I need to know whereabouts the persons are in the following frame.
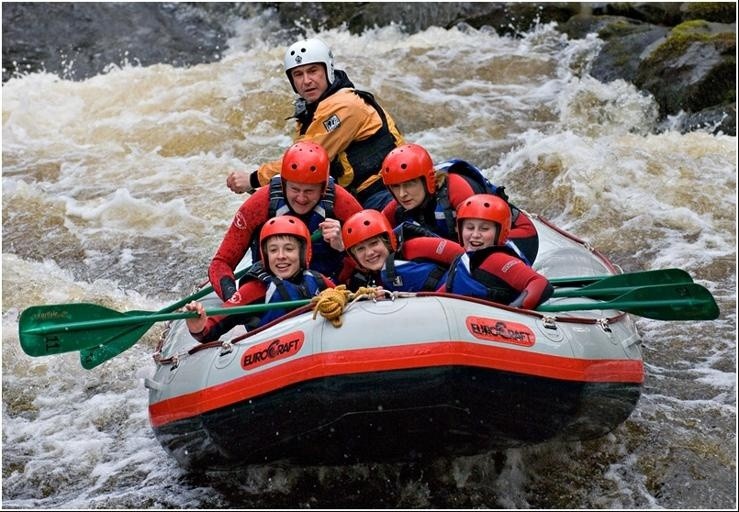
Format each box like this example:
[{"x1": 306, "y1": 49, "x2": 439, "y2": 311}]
[
  {"x1": 434, "y1": 194, "x2": 554, "y2": 311},
  {"x1": 181, "y1": 216, "x2": 338, "y2": 344},
  {"x1": 208, "y1": 142, "x2": 364, "y2": 302},
  {"x1": 341, "y1": 210, "x2": 447, "y2": 292},
  {"x1": 226, "y1": 38, "x2": 402, "y2": 214},
  {"x1": 379, "y1": 144, "x2": 538, "y2": 267}
]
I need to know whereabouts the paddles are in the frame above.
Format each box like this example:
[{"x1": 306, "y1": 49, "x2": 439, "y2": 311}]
[
  {"x1": 80, "y1": 231, "x2": 320, "y2": 370},
  {"x1": 534, "y1": 283, "x2": 721, "y2": 321},
  {"x1": 18, "y1": 299, "x2": 308, "y2": 357},
  {"x1": 551, "y1": 269, "x2": 693, "y2": 301}
]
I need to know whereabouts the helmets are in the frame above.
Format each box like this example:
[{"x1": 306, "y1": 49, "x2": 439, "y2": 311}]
[
  {"x1": 343, "y1": 209, "x2": 398, "y2": 270},
  {"x1": 456, "y1": 194, "x2": 512, "y2": 246},
  {"x1": 382, "y1": 144, "x2": 436, "y2": 201},
  {"x1": 281, "y1": 142, "x2": 330, "y2": 198},
  {"x1": 259, "y1": 216, "x2": 314, "y2": 270},
  {"x1": 285, "y1": 39, "x2": 335, "y2": 94}
]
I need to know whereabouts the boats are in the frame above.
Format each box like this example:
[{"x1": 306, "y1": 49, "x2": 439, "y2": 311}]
[{"x1": 145, "y1": 207, "x2": 644, "y2": 471}]
